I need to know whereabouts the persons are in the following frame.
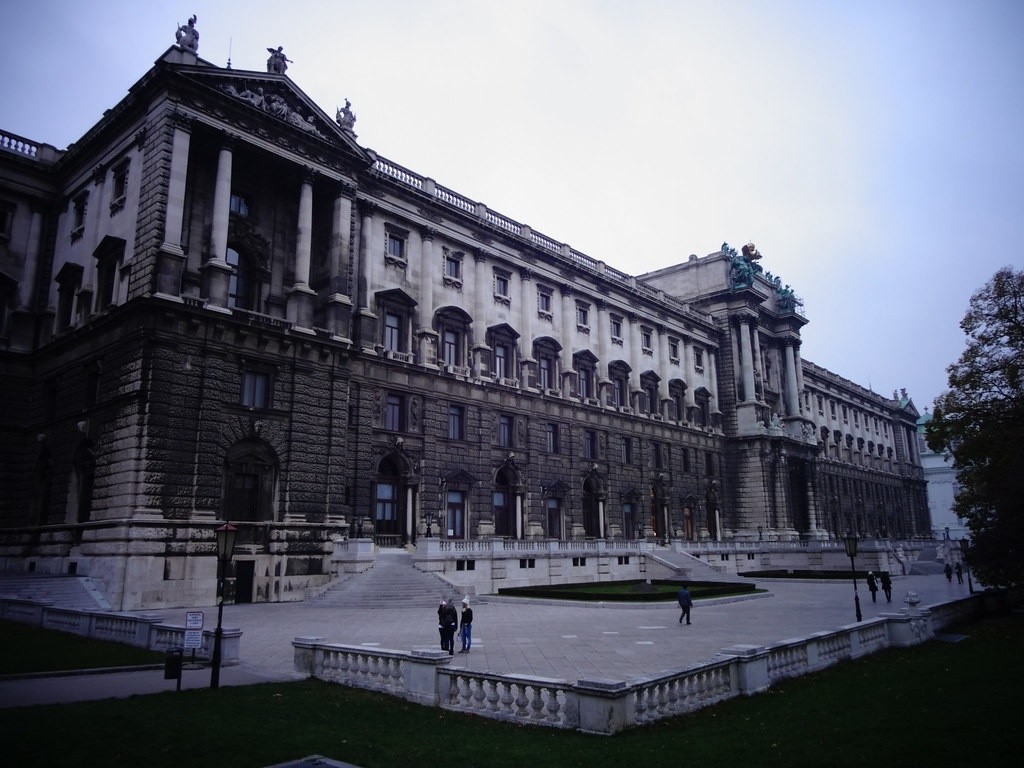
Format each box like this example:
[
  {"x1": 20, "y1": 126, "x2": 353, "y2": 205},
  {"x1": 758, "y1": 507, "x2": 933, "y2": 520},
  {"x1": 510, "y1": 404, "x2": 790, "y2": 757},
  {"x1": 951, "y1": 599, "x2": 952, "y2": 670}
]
[
  {"x1": 881, "y1": 571, "x2": 892, "y2": 603},
  {"x1": 773, "y1": 285, "x2": 802, "y2": 311},
  {"x1": 944, "y1": 564, "x2": 952, "y2": 582},
  {"x1": 337, "y1": 97, "x2": 356, "y2": 128},
  {"x1": 176, "y1": 14, "x2": 199, "y2": 51},
  {"x1": 678, "y1": 584, "x2": 693, "y2": 625},
  {"x1": 867, "y1": 571, "x2": 879, "y2": 603},
  {"x1": 954, "y1": 562, "x2": 963, "y2": 584},
  {"x1": 266, "y1": 46, "x2": 293, "y2": 74},
  {"x1": 729, "y1": 257, "x2": 759, "y2": 285},
  {"x1": 239, "y1": 88, "x2": 320, "y2": 135},
  {"x1": 437, "y1": 598, "x2": 458, "y2": 655},
  {"x1": 458, "y1": 597, "x2": 473, "y2": 653},
  {"x1": 893, "y1": 389, "x2": 899, "y2": 400}
]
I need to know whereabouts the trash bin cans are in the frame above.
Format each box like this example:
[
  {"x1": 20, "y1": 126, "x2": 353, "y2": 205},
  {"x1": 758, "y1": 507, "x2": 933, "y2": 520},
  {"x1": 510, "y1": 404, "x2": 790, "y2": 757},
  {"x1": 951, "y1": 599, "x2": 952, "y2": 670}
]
[{"x1": 163, "y1": 646, "x2": 184, "y2": 680}]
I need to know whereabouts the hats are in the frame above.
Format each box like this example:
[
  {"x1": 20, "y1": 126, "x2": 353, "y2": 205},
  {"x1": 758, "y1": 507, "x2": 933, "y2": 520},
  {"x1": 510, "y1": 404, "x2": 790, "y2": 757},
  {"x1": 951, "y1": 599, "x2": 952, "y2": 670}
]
[
  {"x1": 462, "y1": 596, "x2": 469, "y2": 604},
  {"x1": 440, "y1": 599, "x2": 446, "y2": 605},
  {"x1": 447, "y1": 598, "x2": 452, "y2": 606},
  {"x1": 868, "y1": 570, "x2": 873, "y2": 575}
]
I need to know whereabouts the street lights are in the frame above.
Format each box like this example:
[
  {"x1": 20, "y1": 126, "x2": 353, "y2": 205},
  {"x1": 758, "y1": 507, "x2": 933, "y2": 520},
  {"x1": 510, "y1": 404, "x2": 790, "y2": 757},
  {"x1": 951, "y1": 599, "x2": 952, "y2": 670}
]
[
  {"x1": 840, "y1": 533, "x2": 872, "y2": 622},
  {"x1": 955, "y1": 535, "x2": 980, "y2": 594},
  {"x1": 210, "y1": 518, "x2": 238, "y2": 691}
]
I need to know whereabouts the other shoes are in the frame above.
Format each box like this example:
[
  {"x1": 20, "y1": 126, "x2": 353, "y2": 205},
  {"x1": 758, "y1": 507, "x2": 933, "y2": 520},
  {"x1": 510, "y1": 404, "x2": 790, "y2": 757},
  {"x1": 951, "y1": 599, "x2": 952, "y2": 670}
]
[
  {"x1": 458, "y1": 650, "x2": 470, "y2": 653},
  {"x1": 680, "y1": 621, "x2": 682, "y2": 623},
  {"x1": 887, "y1": 600, "x2": 892, "y2": 603},
  {"x1": 687, "y1": 623, "x2": 691, "y2": 624}
]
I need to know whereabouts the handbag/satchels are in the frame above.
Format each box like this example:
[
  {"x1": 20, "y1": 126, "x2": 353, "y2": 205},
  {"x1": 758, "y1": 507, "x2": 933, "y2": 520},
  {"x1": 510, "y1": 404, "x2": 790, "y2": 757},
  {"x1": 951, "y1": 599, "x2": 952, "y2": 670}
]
[
  {"x1": 457, "y1": 633, "x2": 462, "y2": 642},
  {"x1": 874, "y1": 580, "x2": 880, "y2": 589}
]
